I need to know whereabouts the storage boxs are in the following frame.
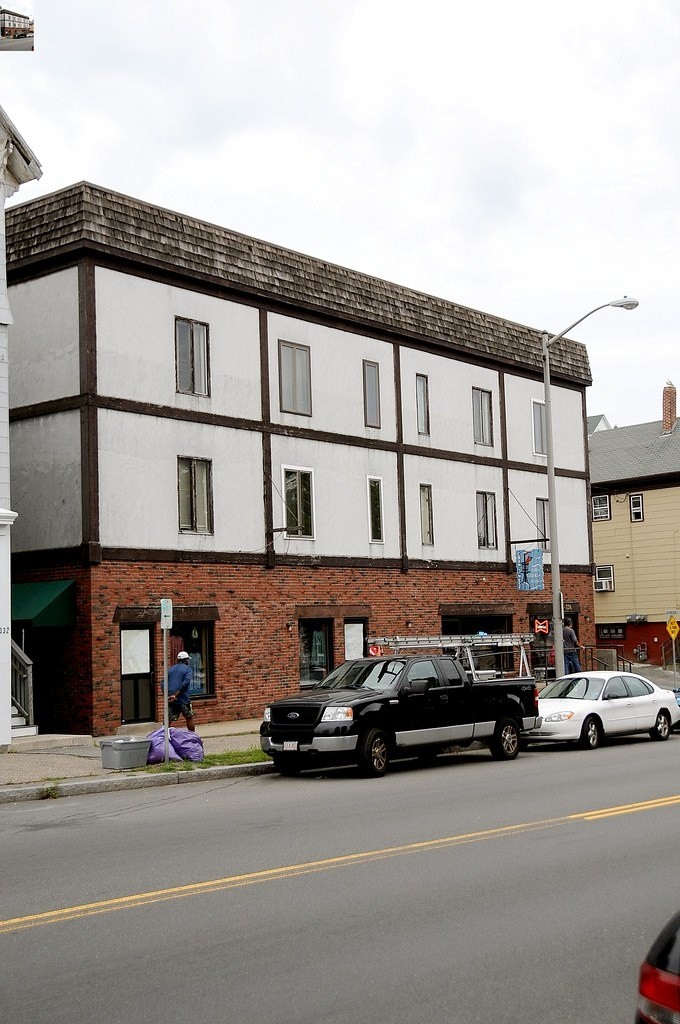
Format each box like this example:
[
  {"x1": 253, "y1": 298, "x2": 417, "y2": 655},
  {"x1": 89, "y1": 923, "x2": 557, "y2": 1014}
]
[{"x1": 99, "y1": 735, "x2": 153, "y2": 769}]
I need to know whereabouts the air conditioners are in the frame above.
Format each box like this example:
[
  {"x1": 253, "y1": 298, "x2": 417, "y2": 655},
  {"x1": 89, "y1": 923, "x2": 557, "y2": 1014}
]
[{"x1": 593, "y1": 580, "x2": 609, "y2": 590}]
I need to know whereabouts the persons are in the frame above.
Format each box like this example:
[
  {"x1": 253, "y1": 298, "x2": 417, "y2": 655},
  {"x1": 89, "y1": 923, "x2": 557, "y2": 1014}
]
[
  {"x1": 161, "y1": 651, "x2": 196, "y2": 734},
  {"x1": 563, "y1": 617, "x2": 585, "y2": 676}
]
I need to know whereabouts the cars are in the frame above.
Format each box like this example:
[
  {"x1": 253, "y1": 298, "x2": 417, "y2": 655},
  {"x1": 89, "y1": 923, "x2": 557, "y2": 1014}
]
[{"x1": 525, "y1": 669, "x2": 680, "y2": 750}]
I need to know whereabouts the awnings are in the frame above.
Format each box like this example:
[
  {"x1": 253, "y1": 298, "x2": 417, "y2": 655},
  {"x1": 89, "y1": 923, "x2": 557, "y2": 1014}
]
[{"x1": 12, "y1": 579, "x2": 78, "y2": 628}]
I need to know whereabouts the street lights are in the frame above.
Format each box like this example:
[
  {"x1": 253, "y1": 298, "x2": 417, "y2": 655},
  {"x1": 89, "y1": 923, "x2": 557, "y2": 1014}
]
[{"x1": 539, "y1": 297, "x2": 639, "y2": 679}]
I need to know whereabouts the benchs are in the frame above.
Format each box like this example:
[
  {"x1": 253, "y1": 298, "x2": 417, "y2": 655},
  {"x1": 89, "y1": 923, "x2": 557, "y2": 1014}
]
[
  {"x1": 534, "y1": 667, "x2": 555, "y2": 681},
  {"x1": 495, "y1": 671, "x2": 507, "y2": 679}
]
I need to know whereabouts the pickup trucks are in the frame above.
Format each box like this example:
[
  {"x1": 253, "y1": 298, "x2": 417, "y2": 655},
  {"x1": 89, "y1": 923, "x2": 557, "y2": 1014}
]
[{"x1": 259, "y1": 632, "x2": 544, "y2": 780}]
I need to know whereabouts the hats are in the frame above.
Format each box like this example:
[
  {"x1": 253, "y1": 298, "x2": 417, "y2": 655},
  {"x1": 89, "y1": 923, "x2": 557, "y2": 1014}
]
[{"x1": 177, "y1": 651, "x2": 192, "y2": 659}]
